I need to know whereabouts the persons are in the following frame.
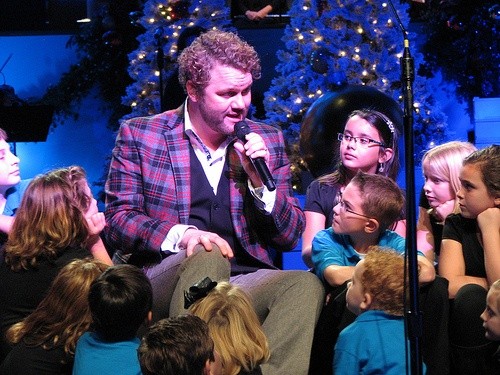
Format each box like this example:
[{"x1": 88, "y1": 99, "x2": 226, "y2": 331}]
[
  {"x1": 245, "y1": 0, "x2": 282, "y2": 20},
  {"x1": 0, "y1": 28, "x2": 500, "y2": 375}
]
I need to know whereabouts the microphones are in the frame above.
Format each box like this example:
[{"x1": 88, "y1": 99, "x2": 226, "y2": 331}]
[{"x1": 234, "y1": 120, "x2": 276, "y2": 192}]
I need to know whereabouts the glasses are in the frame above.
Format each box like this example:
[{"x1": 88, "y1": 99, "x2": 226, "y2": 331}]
[
  {"x1": 337, "y1": 132, "x2": 384, "y2": 147},
  {"x1": 336, "y1": 191, "x2": 371, "y2": 220}
]
